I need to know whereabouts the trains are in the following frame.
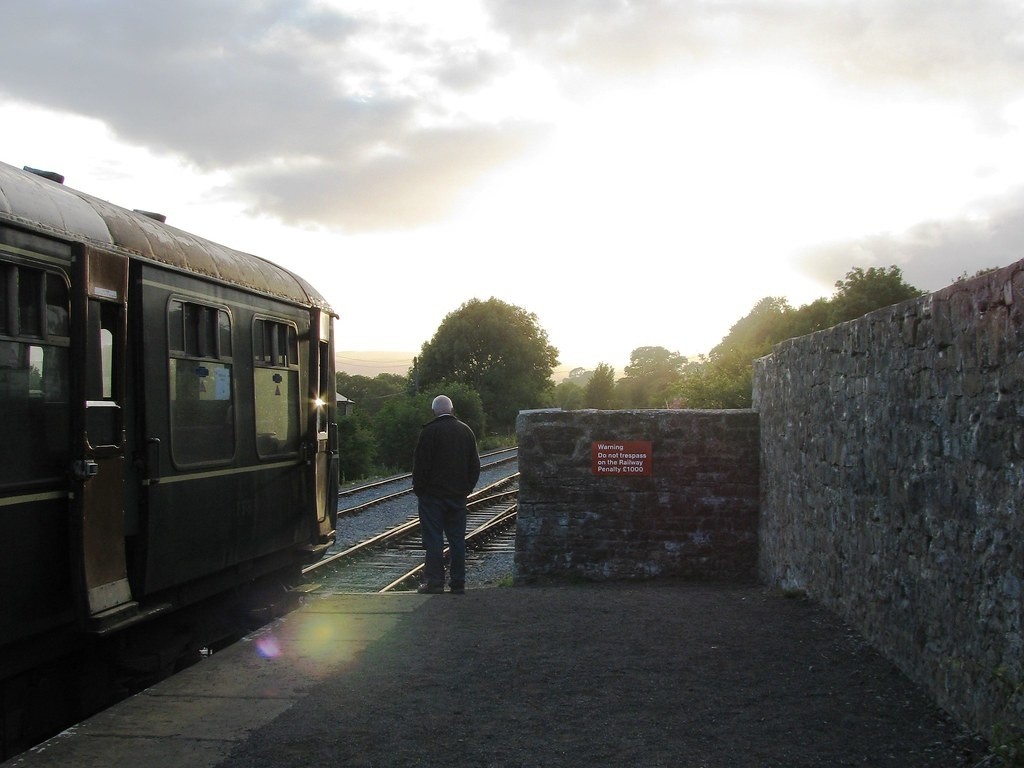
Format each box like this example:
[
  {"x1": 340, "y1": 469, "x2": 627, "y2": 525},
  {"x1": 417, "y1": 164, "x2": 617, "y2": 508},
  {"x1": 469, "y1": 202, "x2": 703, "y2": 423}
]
[{"x1": 1, "y1": 159, "x2": 357, "y2": 681}]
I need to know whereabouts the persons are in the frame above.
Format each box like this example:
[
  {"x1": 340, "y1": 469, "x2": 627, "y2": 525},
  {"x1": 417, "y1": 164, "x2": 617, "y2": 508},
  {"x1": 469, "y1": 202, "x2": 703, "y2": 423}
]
[{"x1": 412, "y1": 395, "x2": 480, "y2": 594}]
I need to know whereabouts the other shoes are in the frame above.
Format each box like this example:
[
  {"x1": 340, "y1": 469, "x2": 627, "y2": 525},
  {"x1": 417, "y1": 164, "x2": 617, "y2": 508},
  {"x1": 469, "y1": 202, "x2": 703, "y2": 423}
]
[
  {"x1": 449, "y1": 581, "x2": 465, "y2": 594},
  {"x1": 418, "y1": 584, "x2": 444, "y2": 594}
]
